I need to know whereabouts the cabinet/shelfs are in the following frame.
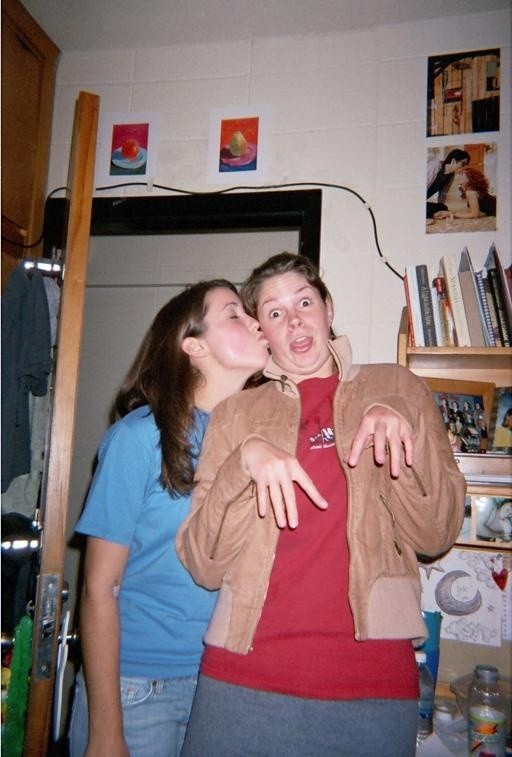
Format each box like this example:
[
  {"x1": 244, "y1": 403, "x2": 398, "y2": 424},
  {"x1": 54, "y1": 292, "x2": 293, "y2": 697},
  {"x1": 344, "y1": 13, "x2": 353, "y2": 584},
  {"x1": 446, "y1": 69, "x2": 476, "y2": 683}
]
[
  {"x1": 1, "y1": 1, "x2": 65, "y2": 295},
  {"x1": 397, "y1": 307, "x2": 512, "y2": 702}
]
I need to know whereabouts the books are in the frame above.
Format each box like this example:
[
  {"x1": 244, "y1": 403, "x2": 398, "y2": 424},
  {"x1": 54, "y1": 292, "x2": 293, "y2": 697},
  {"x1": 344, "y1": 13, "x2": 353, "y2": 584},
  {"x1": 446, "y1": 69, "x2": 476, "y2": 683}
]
[{"x1": 403, "y1": 241, "x2": 512, "y2": 347}]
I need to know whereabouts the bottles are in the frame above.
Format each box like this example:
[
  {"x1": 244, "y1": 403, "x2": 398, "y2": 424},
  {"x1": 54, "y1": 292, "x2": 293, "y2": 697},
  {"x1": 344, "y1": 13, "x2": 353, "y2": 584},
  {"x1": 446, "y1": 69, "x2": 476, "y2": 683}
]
[
  {"x1": 416, "y1": 651, "x2": 435, "y2": 747},
  {"x1": 468, "y1": 664, "x2": 506, "y2": 757}
]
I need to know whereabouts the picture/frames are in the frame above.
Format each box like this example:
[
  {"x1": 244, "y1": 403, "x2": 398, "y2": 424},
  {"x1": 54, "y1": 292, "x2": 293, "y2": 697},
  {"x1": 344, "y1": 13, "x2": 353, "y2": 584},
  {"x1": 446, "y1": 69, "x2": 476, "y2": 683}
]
[
  {"x1": 486, "y1": 387, "x2": 512, "y2": 451},
  {"x1": 419, "y1": 377, "x2": 495, "y2": 453}
]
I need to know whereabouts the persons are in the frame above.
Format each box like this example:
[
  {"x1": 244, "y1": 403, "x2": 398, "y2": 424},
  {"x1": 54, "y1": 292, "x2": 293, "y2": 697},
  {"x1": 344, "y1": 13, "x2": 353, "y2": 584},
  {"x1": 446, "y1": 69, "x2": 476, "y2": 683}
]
[
  {"x1": 439, "y1": 398, "x2": 487, "y2": 453},
  {"x1": 69, "y1": 279, "x2": 270, "y2": 757},
  {"x1": 435, "y1": 167, "x2": 496, "y2": 218},
  {"x1": 426, "y1": 149, "x2": 471, "y2": 219},
  {"x1": 175, "y1": 250, "x2": 466, "y2": 757},
  {"x1": 475, "y1": 497, "x2": 512, "y2": 542}
]
